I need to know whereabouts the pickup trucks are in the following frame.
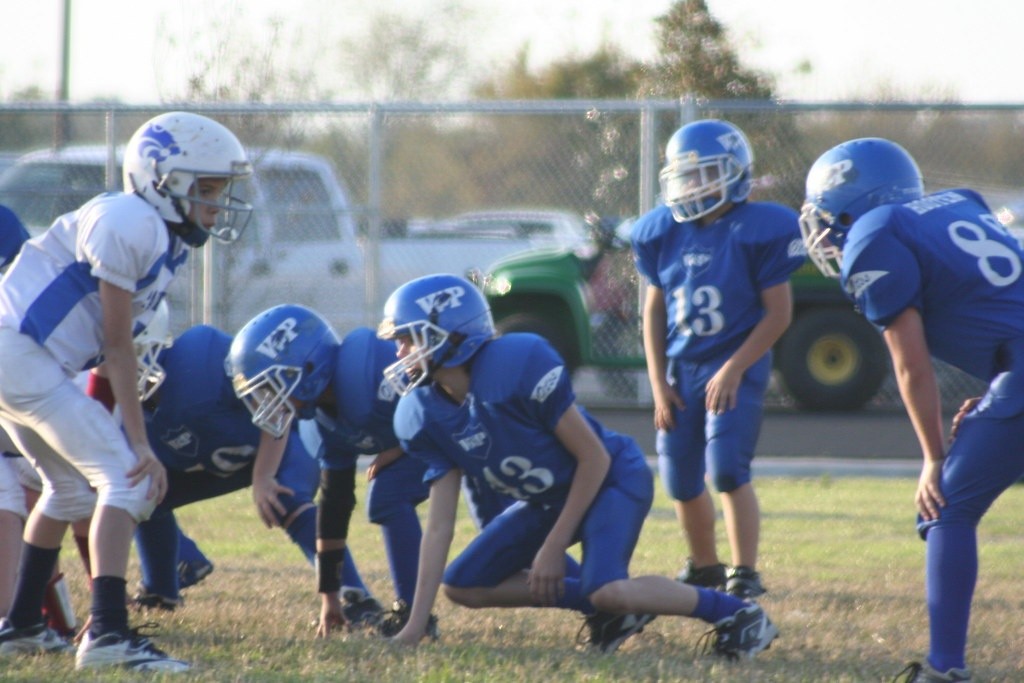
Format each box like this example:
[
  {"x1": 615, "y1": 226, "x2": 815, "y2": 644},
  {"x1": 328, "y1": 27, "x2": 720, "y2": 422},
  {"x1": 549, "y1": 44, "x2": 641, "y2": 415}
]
[{"x1": 0, "y1": 146, "x2": 589, "y2": 353}]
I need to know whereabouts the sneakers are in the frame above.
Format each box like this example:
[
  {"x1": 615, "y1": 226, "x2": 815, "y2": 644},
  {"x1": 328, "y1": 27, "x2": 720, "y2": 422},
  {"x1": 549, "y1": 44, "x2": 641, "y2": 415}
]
[
  {"x1": 73, "y1": 626, "x2": 192, "y2": 675},
  {"x1": 575, "y1": 609, "x2": 656, "y2": 659},
  {"x1": 335, "y1": 595, "x2": 382, "y2": 631},
  {"x1": 724, "y1": 564, "x2": 763, "y2": 598},
  {"x1": 366, "y1": 603, "x2": 437, "y2": 642},
  {"x1": 178, "y1": 556, "x2": 213, "y2": 588},
  {"x1": 128, "y1": 580, "x2": 182, "y2": 615},
  {"x1": 693, "y1": 598, "x2": 779, "y2": 668},
  {"x1": 674, "y1": 558, "x2": 728, "y2": 587},
  {"x1": 0, "y1": 613, "x2": 69, "y2": 656}
]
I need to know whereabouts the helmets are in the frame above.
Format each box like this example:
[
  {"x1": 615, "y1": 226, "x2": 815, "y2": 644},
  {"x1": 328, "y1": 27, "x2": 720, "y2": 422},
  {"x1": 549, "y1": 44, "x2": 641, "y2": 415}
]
[
  {"x1": 93, "y1": 296, "x2": 171, "y2": 402},
  {"x1": 657, "y1": 118, "x2": 753, "y2": 222},
  {"x1": 798, "y1": 137, "x2": 924, "y2": 276},
  {"x1": 223, "y1": 303, "x2": 341, "y2": 440},
  {"x1": 377, "y1": 273, "x2": 497, "y2": 398},
  {"x1": 123, "y1": 113, "x2": 255, "y2": 247}
]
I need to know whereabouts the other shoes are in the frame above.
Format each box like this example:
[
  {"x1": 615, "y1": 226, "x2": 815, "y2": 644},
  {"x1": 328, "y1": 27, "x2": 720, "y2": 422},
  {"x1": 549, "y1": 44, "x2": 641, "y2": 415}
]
[{"x1": 889, "y1": 661, "x2": 969, "y2": 683}]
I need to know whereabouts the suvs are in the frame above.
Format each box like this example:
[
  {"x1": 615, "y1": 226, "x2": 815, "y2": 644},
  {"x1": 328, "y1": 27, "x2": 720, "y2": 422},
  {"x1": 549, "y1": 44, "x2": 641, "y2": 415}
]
[{"x1": 465, "y1": 207, "x2": 895, "y2": 416}]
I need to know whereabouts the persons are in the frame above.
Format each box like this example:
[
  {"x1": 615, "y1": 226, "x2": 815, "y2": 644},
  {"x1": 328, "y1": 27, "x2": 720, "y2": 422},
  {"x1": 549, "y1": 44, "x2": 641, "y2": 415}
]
[
  {"x1": 0, "y1": 113, "x2": 255, "y2": 672},
  {"x1": 630, "y1": 120, "x2": 806, "y2": 599},
  {"x1": 377, "y1": 273, "x2": 780, "y2": 672},
  {"x1": 797, "y1": 137, "x2": 1024, "y2": 683},
  {"x1": 224, "y1": 304, "x2": 583, "y2": 643},
  {"x1": 128, "y1": 295, "x2": 383, "y2": 633}
]
